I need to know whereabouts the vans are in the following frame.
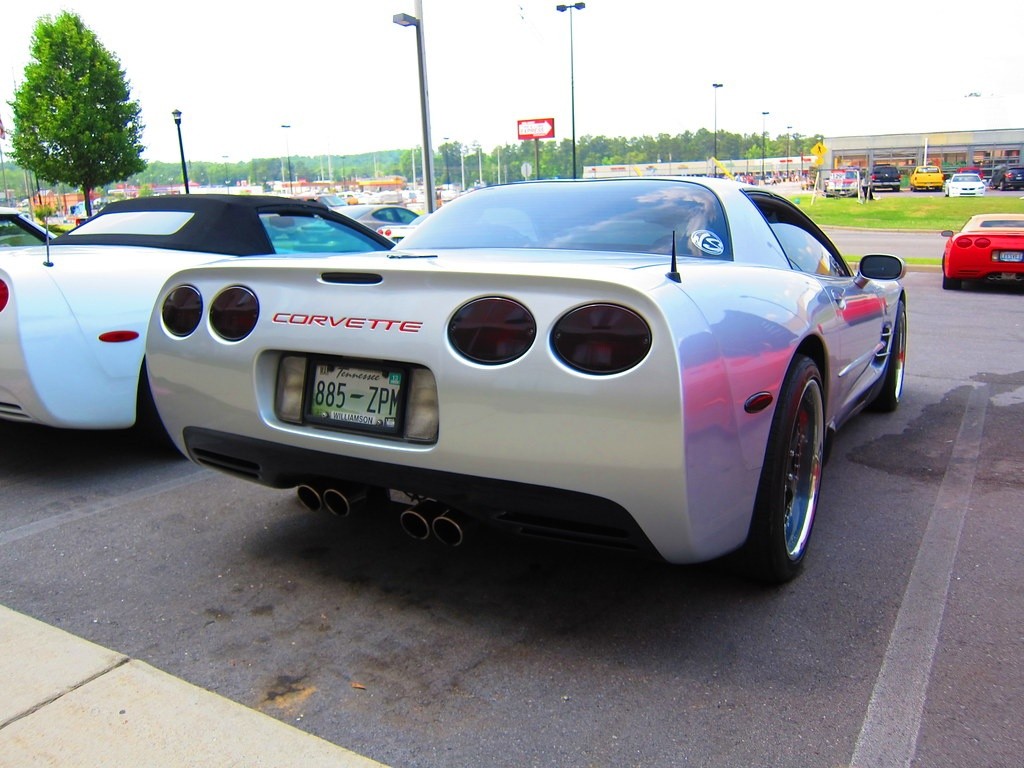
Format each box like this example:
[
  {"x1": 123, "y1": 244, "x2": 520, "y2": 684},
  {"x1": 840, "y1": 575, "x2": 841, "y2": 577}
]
[{"x1": 957, "y1": 164, "x2": 984, "y2": 182}]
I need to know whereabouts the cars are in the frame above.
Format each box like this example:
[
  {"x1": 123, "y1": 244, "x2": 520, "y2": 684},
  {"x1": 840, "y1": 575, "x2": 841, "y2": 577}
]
[
  {"x1": 0, "y1": 167, "x2": 472, "y2": 279},
  {"x1": 824, "y1": 167, "x2": 863, "y2": 197},
  {"x1": 910, "y1": 165, "x2": 945, "y2": 192},
  {"x1": 989, "y1": 163, "x2": 1024, "y2": 191},
  {"x1": 944, "y1": 173, "x2": 986, "y2": 199}
]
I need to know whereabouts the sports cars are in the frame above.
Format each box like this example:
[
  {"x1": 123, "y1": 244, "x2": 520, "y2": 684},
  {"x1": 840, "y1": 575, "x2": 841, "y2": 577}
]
[
  {"x1": 0, "y1": 191, "x2": 396, "y2": 435},
  {"x1": 941, "y1": 214, "x2": 1024, "y2": 290},
  {"x1": 147, "y1": 172, "x2": 908, "y2": 589}
]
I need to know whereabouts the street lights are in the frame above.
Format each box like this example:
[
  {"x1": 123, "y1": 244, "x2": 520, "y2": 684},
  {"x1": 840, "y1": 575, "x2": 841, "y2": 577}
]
[
  {"x1": 171, "y1": 108, "x2": 190, "y2": 194},
  {"x1": 392, "y1": 0, "x2": 437, "y2": 215},
  {"x1": 761, "y1": 112, "x2": 770, "y2": 180},
  {"x1": 712, "y1": 83, "x2": 724, "y2": 178},
  {"x1": 557, "y1": 2, "x2": 587, "y2": 179}
]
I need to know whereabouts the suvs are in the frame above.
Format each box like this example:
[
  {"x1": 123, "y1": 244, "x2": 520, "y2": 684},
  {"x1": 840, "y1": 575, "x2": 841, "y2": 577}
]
[{"x1": 870, "y1": 166, "x2": 901, "y2": 192}]
